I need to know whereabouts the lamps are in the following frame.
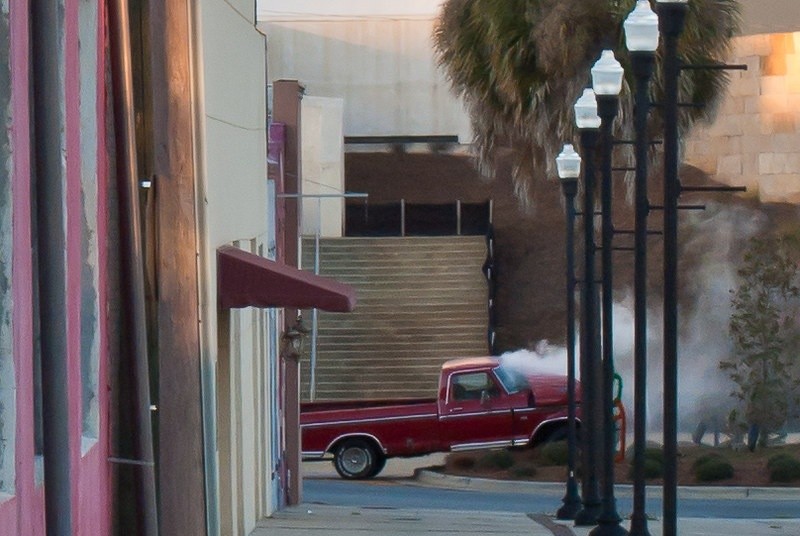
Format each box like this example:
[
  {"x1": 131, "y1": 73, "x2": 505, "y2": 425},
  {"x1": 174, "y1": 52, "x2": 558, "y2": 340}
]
[{"x1": 281, "y1": 316, "x2": 312, "y2": 367}]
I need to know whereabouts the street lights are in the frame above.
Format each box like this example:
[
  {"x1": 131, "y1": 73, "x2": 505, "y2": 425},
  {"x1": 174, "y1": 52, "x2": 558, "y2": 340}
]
[
  {"x1": 589, "y1": 48, "x2": 630, "y2": 536},
  {"x1": 623, "y1": 0, "x2": 660, "y2": 536},
  {"x1": 555, "y1": 142, "x2": 584, "y2": 522},
  {"x1": 574, "y1": 86, "x2": 602, "y2": 527}
]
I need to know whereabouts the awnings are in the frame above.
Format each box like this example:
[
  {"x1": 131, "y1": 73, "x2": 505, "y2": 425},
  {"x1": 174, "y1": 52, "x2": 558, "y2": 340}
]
[{"x1": 216, "y1": 244, "x2": 357, "y2": 313}]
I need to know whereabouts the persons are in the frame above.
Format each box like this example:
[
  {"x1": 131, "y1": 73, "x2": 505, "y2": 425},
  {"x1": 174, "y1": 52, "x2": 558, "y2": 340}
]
[{"x1": 748, "y1": 417, "x2": 760, "y2": 451}]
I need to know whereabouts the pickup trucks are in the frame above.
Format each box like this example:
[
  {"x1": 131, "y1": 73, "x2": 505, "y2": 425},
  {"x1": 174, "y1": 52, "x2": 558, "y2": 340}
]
[{"x1": 299, "y1": 356, "x2": 581, "y2": 482}]
